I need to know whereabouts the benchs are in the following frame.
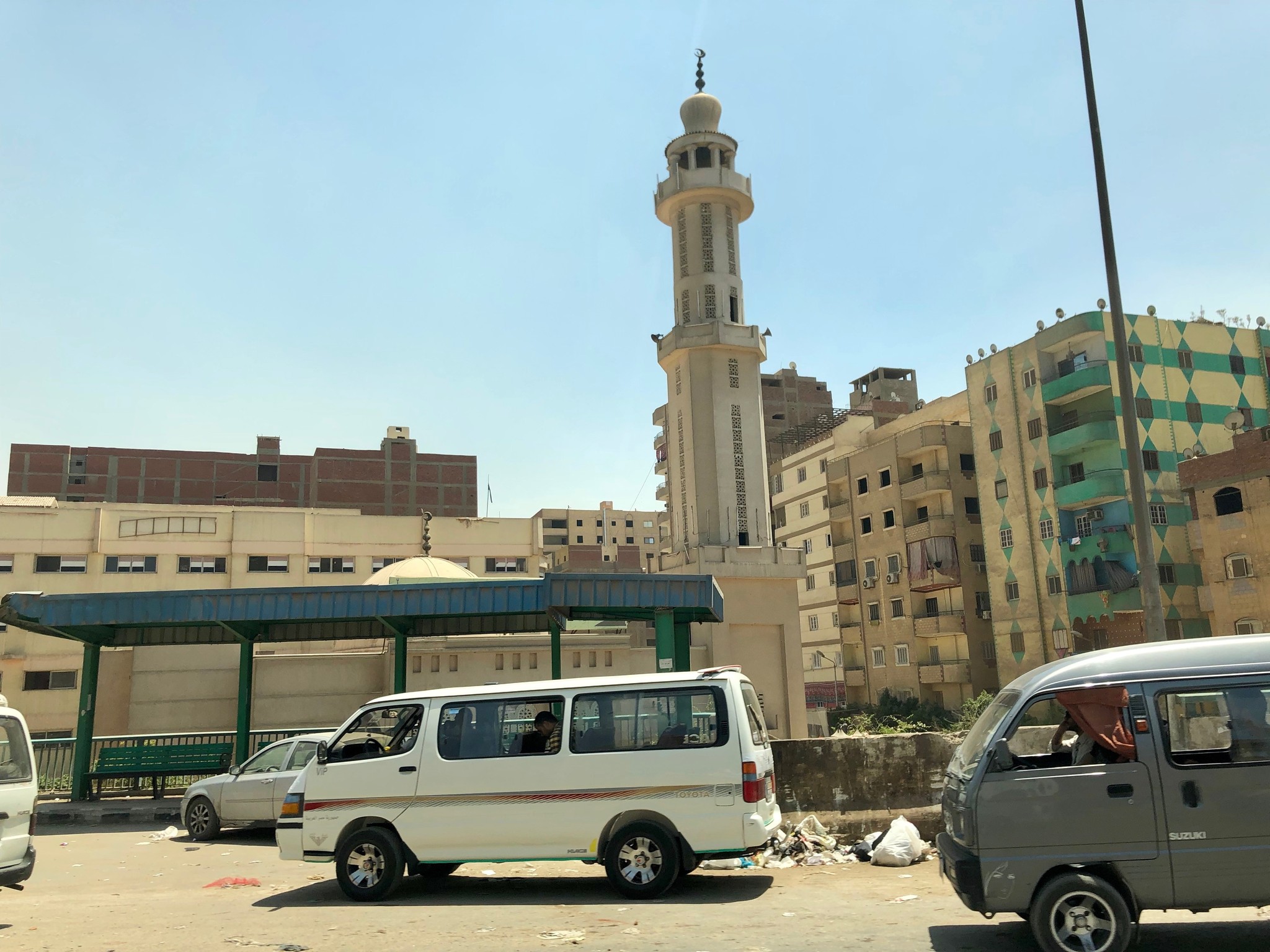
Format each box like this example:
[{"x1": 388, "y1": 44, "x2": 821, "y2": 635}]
[
  {"x1": 254, "y1": 741, "x2": 317, "y2": 768},
  {"x1": 83, "y1": 742, "x2": 235, "y2": 803}
]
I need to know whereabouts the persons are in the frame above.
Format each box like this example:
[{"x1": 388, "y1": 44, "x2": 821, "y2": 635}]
[
  {"x1": 1052, "y1": 710, "x2": 1096, "y2": 766},
  {"x1": 534, "y1": 711, "x2": 562, "y2": 752},
  {"x1": 444, "y1": 707, "x2": 480, "y2": 746}
]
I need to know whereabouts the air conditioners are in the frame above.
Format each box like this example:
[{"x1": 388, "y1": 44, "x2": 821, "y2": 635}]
[
  {"x1": 885, "y1": 573, "x2": 898, "y2": 584},
  {"x1": 862, "y1": 578, "x2": 875, "y2": 590},
  {"x1": 975, "y1": 564, "x2": 986, "y2": 573},
  {"x1": 1086, "y1": 510, "x2": 1104, "y2": 521},
  {"x1": 983, "y1": 611, "x2": 992, "y2": 620}
]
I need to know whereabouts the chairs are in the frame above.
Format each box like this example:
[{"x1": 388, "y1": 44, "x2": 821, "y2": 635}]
[
  {"x1": 600, "y1": 726, "x2": 617, "y2": 751},
  {"x1": 506, "y1": 729, "x2": 541, "y2": 755},
  {"x1": 655, "y1": 723, "x2": 688, "y2": 748},
  {"x1": 687, "y1": 725, "x2": 703, "y2": 744},
  {"x1": 441, "y1": 721, "x2": 480, "y2": 758},
  {"x1": 575, "y1": 726, "x2": 603, "y2": 751}
]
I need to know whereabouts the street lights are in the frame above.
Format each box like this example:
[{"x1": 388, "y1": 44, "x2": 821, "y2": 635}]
[{"x1": 816, "y1": 649, "x2": 838, "y2": 708}]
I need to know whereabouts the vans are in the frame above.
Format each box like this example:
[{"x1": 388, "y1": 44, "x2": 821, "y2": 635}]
[
  {"x1": 0, "y1": 694, "x2": 40, "y2": 892},
  {"x1": 934, "y1": 631, "x2": 1270, "y2": 952},
  {"x1": 275, "y1": 663, "x2": 785, "y2": 903}
]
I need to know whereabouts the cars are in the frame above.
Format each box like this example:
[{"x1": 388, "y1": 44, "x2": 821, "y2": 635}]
[{"x1": 179, "y1": 730, "x2": 395, "y2": 841}]
